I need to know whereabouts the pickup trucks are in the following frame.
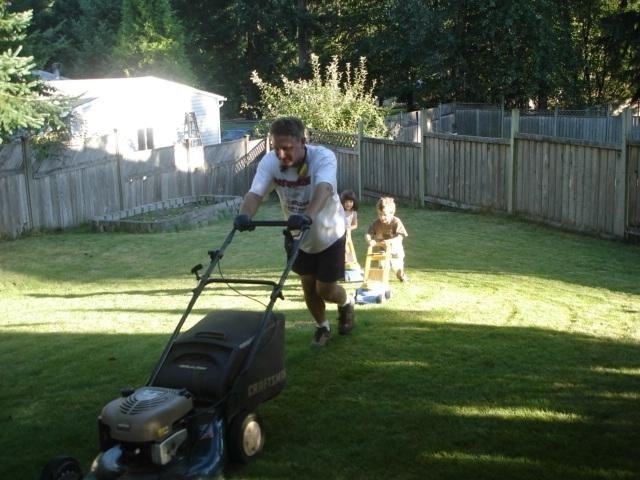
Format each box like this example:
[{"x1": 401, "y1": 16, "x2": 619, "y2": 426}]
[{"x1": 221, "y1": 128, "x2": 263, "y2": 142}]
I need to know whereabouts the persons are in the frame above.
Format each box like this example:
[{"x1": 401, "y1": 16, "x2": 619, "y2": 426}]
[
  {"x1": 339, "y1": 188, "x2": 358, "y2": 260},
  {"x1": 364, "y1": 197, "x2": 408, "y2": 281},
  {"x1": 234, "y1": 116, "x2": 355, "y2": 349}
]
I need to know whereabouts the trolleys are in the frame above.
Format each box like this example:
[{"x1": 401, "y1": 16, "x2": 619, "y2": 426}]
[{"x1": 40, "y1": 216, "x2": 314, "y2": 479}]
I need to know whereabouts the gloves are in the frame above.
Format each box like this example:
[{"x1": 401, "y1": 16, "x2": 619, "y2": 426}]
[
  {"x1": 287, "y1": 213, "x2": 313, "y2": 231},
  {"x1": 233, "y1": 214, "x2": 256, "y2": 232}
]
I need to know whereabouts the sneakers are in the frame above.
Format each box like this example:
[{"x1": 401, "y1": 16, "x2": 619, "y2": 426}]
[
  {"x1": 396, "y1": 269, "x2": 406, "y2": 281},
  {"x1": 309, "y1": 325, "x2": 332, "y2": 349},
  {"x1": 337, "y1": 293, "x2": 356, "y2": 336}
]
